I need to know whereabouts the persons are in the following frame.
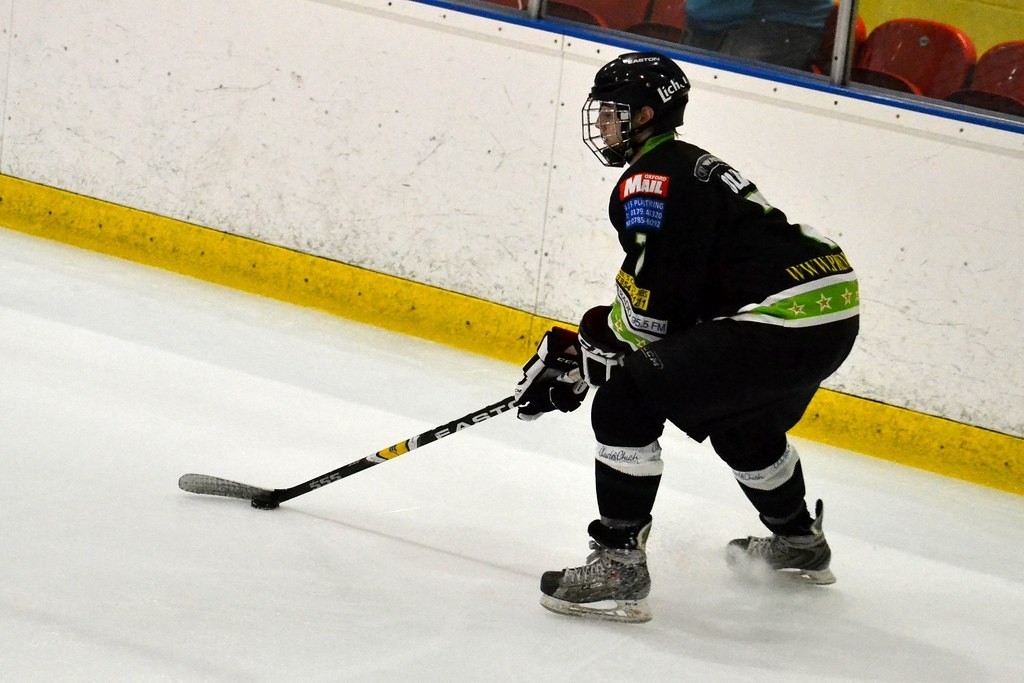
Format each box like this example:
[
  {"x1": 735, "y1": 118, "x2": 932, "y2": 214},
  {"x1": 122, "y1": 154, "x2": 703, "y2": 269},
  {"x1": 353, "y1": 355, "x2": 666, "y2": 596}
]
[
  {"x1": 683, "y1": 0, "x2": 834, "y2": 71},
  {"x1": 514, "y1": 50, "x2": 862, "y2": 623}
]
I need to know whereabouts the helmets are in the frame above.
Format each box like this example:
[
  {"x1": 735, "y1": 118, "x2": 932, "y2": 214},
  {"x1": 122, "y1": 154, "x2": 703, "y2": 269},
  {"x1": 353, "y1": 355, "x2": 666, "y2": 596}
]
[{"x1": 582, "y1": 52, "x2": 691, "y2": 167}]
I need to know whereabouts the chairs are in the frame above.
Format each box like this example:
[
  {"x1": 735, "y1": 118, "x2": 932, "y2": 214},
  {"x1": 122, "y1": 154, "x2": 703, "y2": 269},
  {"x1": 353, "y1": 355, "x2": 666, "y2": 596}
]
[
  {"x1": 971, "y1": 40, "x2": 1024, "y2": 103},
  {"x1": 563, "y1": 0, "x2": 649, "y2": 32},
  {"x1": 850, "y1": 66, "x2": 922, "y2": 96},
  {"x1": 858, "y1": 16, "x2": 976, "y2": 99},
  {"x1": 944, "y1": 90, "x2": 1024, "y2": 117}
]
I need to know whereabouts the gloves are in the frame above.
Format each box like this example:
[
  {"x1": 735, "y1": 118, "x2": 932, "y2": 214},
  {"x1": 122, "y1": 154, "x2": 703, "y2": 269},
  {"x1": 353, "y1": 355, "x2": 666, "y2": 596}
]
[
  {"x1": 579, "y1": 305, "x2": 628, "y2": 386},
  {"x1": 515, "y1": 327, "x2": 590, "y2": 420}
]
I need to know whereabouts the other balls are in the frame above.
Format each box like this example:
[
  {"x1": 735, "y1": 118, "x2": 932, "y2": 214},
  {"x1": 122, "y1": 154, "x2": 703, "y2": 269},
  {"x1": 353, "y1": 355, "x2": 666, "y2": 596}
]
[{"x1": 251, "y1": 496, "x2": 280, "y2": 510}]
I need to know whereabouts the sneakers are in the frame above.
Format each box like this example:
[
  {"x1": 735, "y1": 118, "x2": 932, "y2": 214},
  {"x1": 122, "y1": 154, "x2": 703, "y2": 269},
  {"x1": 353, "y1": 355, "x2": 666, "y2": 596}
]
[
  {"x1": 539, "y1": 514, "x2": 654, "y2": 624},
  {"x1": 723, "y1": 498, "x2": 837, "y2": 586}
]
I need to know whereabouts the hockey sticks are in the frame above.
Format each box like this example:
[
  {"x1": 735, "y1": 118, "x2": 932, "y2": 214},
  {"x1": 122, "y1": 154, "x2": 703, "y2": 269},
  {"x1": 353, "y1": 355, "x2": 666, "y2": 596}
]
[{"x1": 178, "y1": 358, "x2": 585, "y2": 505}]
